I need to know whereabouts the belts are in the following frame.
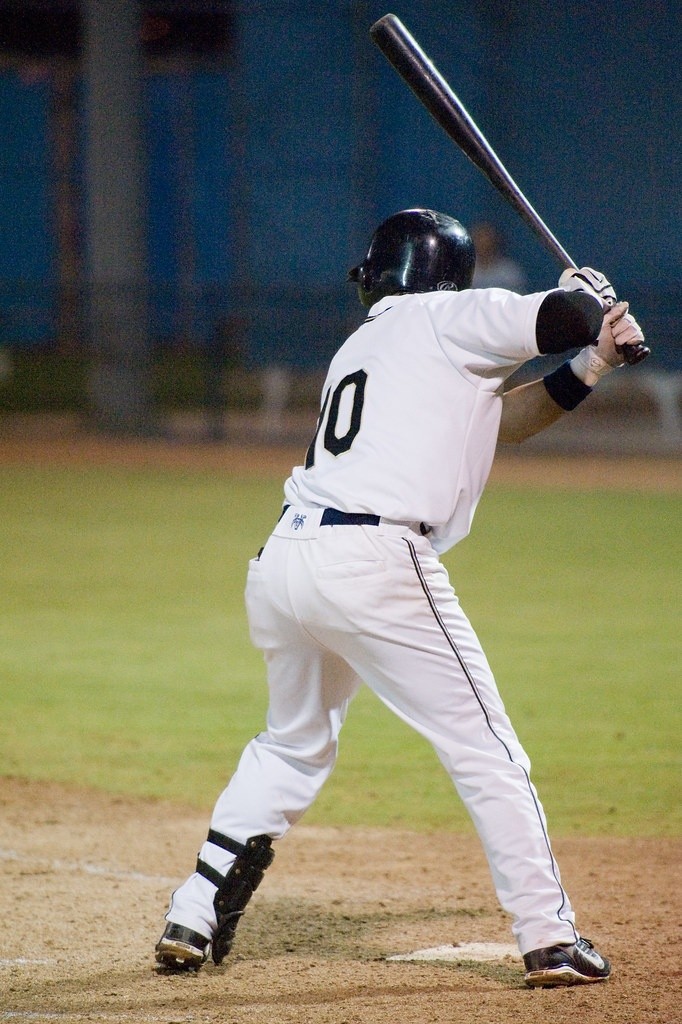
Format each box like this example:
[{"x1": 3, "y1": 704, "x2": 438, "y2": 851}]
[{"x1": 277, "y1": 505, "x2": 428, "y2": 536}]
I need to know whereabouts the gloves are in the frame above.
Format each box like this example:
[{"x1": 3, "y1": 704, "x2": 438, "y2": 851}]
[
  {"x1": 558, "y1": 265, "x2": 617, "y2": 313},
  {"x1": 594, "y1": 301, "x2": 645, "y2": 368}
]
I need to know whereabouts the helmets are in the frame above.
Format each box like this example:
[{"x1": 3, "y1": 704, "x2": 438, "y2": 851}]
[{"x1": 349, "y1": 207, "x2": 476, "y2": 298}]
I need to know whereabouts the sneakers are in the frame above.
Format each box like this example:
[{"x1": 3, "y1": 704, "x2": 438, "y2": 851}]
[
  {"x1": 523, "y1": 937, "x2": 611, "y2": 987},
  {"x1": 155, "y1": 921, "x2": 211, "y2": 972}
]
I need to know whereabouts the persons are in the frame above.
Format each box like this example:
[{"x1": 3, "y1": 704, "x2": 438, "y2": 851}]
[{"x1": 154, "y1": 208, "x2": 646, "y2": 986}]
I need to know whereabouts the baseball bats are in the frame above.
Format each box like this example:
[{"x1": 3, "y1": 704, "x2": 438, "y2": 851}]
[{"x1": 370, "y1": 9, "x2": 656, "y2": 365}]
